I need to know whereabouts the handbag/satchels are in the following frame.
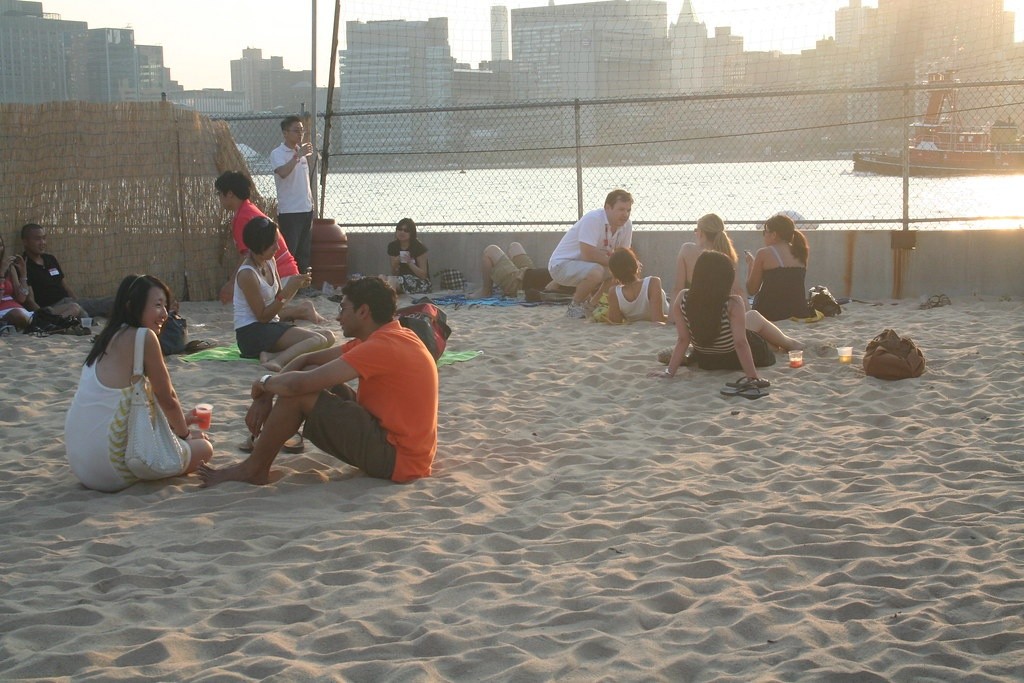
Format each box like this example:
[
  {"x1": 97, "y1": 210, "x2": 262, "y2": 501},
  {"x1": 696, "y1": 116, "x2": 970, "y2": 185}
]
[
  {"x1": 807, "y1": 286, "x2": 841, "y2": 316},
  {"x1": 159, "y1": 301, "x2": 188, "y2": 355},
  {"x1": 863, "y1": 328, "x2": 926, "y2": 380},
  {"x1": 125, "y1": 327, "x2": 186, "y2": 479},
  {"x1": 433, "y1": 269, "x2": 466, "y2": 290}
]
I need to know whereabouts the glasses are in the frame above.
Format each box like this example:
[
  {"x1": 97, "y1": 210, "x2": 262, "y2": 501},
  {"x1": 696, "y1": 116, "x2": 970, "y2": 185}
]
[
  {"x1": 254, "y1": 217, "x2": 273, "y2": 234},
  {"x1": 694, "y1": 228, "x2": 702, "y2": 233},
  {"x1": 396, "y1": 227, "x2": 410, "y2": 233},
  {"x1": 762, "y1": 229, "x2": 766, "y2": 236},
  {"x1": 286, "y1": 127, "x2": 305, "y2": 133}
]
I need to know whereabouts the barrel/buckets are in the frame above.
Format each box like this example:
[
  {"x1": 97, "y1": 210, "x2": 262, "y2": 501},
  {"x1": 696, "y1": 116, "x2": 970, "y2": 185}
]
[{"x1": 311, "y1": 218, "x2": 348, "y2": 289}]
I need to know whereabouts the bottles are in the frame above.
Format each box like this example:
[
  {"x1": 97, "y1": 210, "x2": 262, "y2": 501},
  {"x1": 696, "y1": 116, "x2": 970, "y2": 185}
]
[{"x1": 602, "y1": 245, "x2": 611, "y2": 258}]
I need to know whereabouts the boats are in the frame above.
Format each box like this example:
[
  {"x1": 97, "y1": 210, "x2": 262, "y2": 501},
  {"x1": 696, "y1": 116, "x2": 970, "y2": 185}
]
[{"x1": 848, "y1": 56, "x2": 1024, "y2": 177}]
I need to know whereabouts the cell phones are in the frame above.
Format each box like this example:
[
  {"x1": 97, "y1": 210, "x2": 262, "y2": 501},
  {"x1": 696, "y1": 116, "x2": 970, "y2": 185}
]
[
  {"x1": 744, "y1": 250, "x2": 755, "y2": 261},
  {"x1": 400, "y1": 251, "x2": 409, "y2": 264}
]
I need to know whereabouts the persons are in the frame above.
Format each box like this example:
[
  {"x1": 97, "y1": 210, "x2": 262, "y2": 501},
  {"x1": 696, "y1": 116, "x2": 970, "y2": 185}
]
[
  {"x1": 647, "y1": 249, "x2": 806, "y2": 386},
  {"x1": 466, "y1": 242, "x2": 577, "y2": 303},
  {"x1": 0, "y1": 223, "x2": 98, "y2": 335},
  {"x1": 271, "y1": 116, "x2": 315, "y2": 274},
  {"x1": 379, "y1": 218, "x2": 434, "y2": 296},
  {"x1": 64, "y1": 274, "x2": 214, "y2": 491},
  {"x1": 234, "y1": 217, "x2": 336, "y2": 372},
  {"x1": 608, "y1": 246, "x2": 669, "y2": 323},
  {"x1": 745, "y1": 215, "x2": 808, "y2": 321},
  {"x1": 548, "y1": 189, "x2": 633, "y2": 321},
  {"x1": 216, "y1": 170, "x2": 330, "y2": 325},
  {"x1": 198, "y1": 275, "x2": 439, "y2": 489},
  {"x1": 667, "y1": 213, "x2": 751, "y2": 325}
]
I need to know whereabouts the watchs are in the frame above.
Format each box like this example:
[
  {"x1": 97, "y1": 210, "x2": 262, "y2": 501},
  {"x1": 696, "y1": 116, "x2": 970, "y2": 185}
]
[{"x1": 259, "y1": 374, "x2": 271, "y2": 393}]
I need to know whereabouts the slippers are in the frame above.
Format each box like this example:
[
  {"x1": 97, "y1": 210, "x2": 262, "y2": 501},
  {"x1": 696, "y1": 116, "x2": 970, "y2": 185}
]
[
  {"x1": 238, "y1": 431, "x2": 305, "y2": 454},
  {"x1": 720, "y1": 376, "x2": 770, "y2": 400}
]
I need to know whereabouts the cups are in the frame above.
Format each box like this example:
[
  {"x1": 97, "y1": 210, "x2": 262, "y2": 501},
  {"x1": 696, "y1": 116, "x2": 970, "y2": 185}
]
[
  {"x1": 81, "y1": 318, "x2": 93, "y2": 328},
  {"x1": 517, "y1": 290, "x2": 526, "y2": 301},
  {"x1": 302, "y1": 143, "x2": 312, "y2": 155},
  {"x1": 837, "y1": 347, "x2": 854, "y2": 362},
  {"x1": 788, "y1": 349, "x2": 805, "y2": 368},
  {"x1": 400, "y1": 251, "x2": 409, "y2": 264},
  {"x1": 195, "y1": 403, "x2": 214, "y2": 430}
]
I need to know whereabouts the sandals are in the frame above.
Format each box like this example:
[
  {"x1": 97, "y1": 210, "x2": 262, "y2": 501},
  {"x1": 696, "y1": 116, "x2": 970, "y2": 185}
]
[{"x1": 918, "y1": 294, "x2": 950, "y2": 310}]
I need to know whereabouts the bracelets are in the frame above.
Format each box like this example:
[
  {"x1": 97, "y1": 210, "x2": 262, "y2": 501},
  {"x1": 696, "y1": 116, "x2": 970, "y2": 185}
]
[
  {"x1": 20, "y1": 277, "x2": 28, "y2": 281},
  {"x1": 179, "y1": 429, "x2": 191, "y2": 440},
  {"x1": 18, "y1": 286, "x2": 29, "y2": 296},
  {"x1": 294, "y1": 153, "x2": 301, "y2": 164},
  {"x1": 665, "y1": 368, "x2": 673, "y2": 376},
  {"x1": 275, "y1": 295, "x2": 287, "y2": 304}
]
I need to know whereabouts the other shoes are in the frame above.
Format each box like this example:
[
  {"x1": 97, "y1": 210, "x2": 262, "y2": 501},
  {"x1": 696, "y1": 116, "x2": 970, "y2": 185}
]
[{"x1": 567, "y1": 303, "x2": 586, "y2": 319}]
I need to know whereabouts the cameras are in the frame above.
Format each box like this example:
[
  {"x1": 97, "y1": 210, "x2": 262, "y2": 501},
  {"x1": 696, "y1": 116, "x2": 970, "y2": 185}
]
[{"x1": 8, "y1": 256, "x2": 21, "y2": 264}]
